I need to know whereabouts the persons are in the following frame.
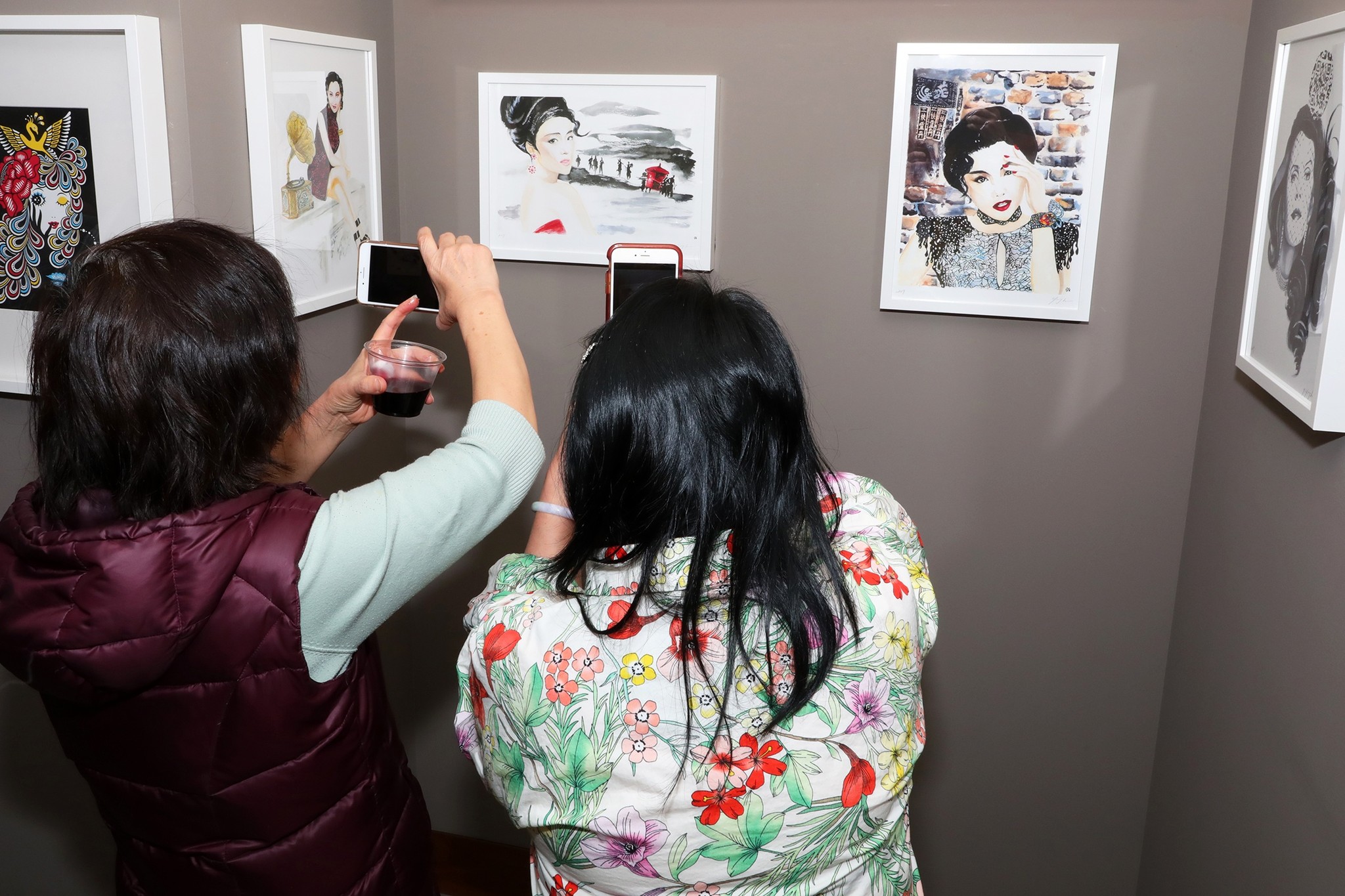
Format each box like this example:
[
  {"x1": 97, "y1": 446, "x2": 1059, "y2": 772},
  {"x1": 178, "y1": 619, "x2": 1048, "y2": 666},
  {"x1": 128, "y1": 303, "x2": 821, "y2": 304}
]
[
  {"x1": 455, "y1": 277, "x2": 938, "y2": 896},
  {"x1": 0, "y1": 219, "x2": 544, "y2": 896}
]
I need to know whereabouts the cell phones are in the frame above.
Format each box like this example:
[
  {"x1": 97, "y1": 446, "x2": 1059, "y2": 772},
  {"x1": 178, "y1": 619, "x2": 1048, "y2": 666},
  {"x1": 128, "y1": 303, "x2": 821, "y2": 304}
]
[
  {"x1": 355, "y1": 239, "x2": 440, "y2": 314},
  {"x1": 604, "y1": 243, "x2": 683, "y2": 324}
]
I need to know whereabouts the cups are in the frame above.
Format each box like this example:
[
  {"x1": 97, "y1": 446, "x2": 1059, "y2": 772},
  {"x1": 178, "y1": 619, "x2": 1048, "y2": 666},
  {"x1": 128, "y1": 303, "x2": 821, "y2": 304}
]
[{"x1": 363, "y1": 339, "x2": 447, "y2": 418}]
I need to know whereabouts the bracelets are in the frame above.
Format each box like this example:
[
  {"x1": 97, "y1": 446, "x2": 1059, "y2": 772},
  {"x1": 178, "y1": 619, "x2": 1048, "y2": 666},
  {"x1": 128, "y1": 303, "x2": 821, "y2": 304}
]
[{"x1": 531, "y1": 501, "x2": 574, "y2": 521}]
